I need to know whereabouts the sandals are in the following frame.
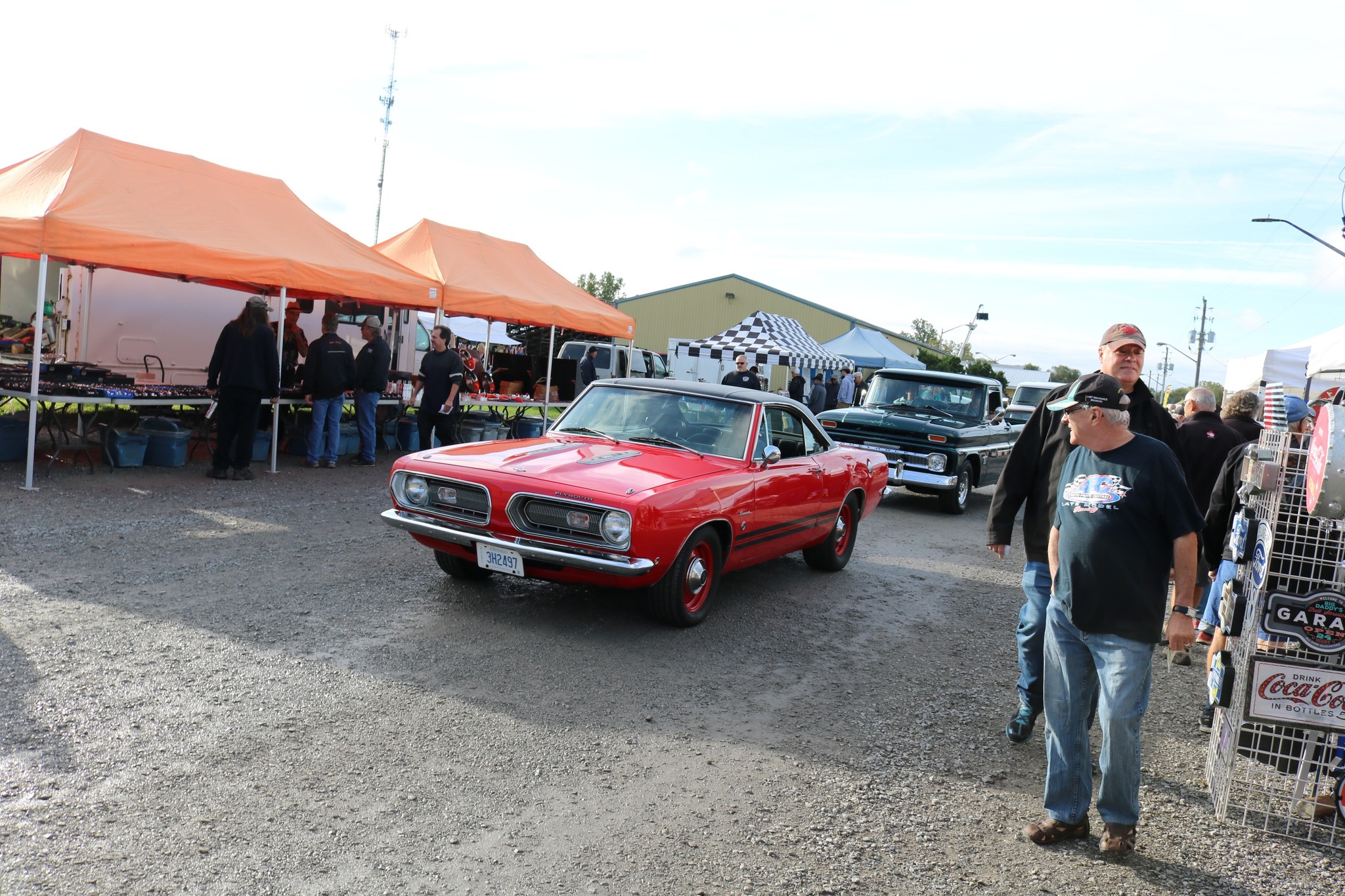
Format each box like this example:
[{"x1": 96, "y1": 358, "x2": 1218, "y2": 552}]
[
  {"x1": 1099, "y1": 822, "x2": 1136, "y2": 857},
  {"x1": 1022, "y1": 811, "x2": 1090, "y2": 845}
]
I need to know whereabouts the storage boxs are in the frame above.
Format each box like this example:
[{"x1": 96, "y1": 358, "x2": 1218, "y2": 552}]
[
  {"x1": 499, "y1": 381, "x2": 558, "y2": 401},
  {"x1": 0, "y1": 411, "x2": 554, "y2": 467},
  {"x1": 28, "y1": 361, "x2": 135, "y2": 386}
]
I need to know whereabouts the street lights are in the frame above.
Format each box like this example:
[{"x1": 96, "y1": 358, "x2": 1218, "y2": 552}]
[
  {"x1": 1157, "y1": 343, "x2": 1200, "y2": 388},
  {"x1": 1140, "y1": 373, "x2": 1164, "y2": 405},
  {"x1": 939, "y1": 324, "x2": 971, "y2": 350}
]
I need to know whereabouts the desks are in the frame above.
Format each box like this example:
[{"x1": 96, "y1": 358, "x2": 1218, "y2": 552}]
[
  {"x1": 262, "y1": 399, "x2": 314, "y2": 457},
  {"x1": 394, "y1": 400, "x2": 572, "y2": 452},
  {"x1": 343, "y1": 399, "x2": 399, "y2": 455},
  {"x1": 0, "y1": 387, "x2": 112, "y2": 478},
  {"x1": 74, "y1": 398, "x2": 214, "y2": 474}
]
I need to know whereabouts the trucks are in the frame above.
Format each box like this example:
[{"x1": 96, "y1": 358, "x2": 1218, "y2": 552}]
[{"x1": 54, "y1": 264, "x2": 432, "y2": 407}]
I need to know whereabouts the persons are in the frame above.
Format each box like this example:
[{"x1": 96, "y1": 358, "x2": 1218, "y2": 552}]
[
  {"x1": 749, "y1": 366, "x2": 759, "y2": 375},
  {"x1": 711, "y1": 404, "x2": 765, "y2": 458},
  {"x1": 204, "y1": 295, "x2": 280, "y2": 481},
  {"x1": 789, "y1": 368, "x2": 806, "y2": 435},
  {"x1": 302, "y1": 314, "x2": 356, "y2": 468},
  {"x1": 269, "y1": 302, "x2": 309, "y2": 373},
  {"x1": 1164, "y1": 387, "x2": 1316, "y2": 733},
  {"x1": 807, "y1": 366, "x2": 951, "y2": 416},
  {"x1": 1025, "y1": 374, "x2": 1207, "y2": 854},
  {"x1": 960, "y1": 387, "x2": 1003, "y2": 421},
  {"x1": 580, "y1": 346, "x2": 598, "y2": 387},
  {"x1": 987, "y1": 324, "x2": 1182, "y2": 744},
  {"x1": 477, "y1": 343, "x2": 485, "y2": 367},
  {"x1": 351, "y1": 315, "x2": 392, "y2": 467},
  {"x1": 715, "y1": 354, "x2": 761, "y2": 425},
  {"x1": 408, "y1": 325, "x2": 464, "y2": 451}
]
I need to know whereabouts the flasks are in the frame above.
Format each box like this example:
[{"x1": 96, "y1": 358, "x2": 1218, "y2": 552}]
[{"x1": 205, "y1": 400, "x2": 218, "y2": 419}]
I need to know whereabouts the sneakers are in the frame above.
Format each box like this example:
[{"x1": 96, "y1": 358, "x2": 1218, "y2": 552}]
[
  {"x1": 1091, "y1": 757, "x2": 1098, "y2": 775},
  {"x1": 1005, "y1": 701, "x2": 1038, "y2": 744},
  {"x1": 206, "y1": 467, "x2": 227, "y2": 479},
  {"x1": 1159, "y1": 647, "x2": 1192, "y2": 665},
  {"x1": 233, "y1": 467, "x2": 255, "y2": 480},
  {"x1": 1198, "y1": 700, "x2": 1215, "y2": 733}
]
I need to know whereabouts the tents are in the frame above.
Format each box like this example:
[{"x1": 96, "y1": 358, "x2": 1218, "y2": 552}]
[
  {"x1": 673, "y1": 310, "x2": 855, "y2": 390},
  {"x1": 0, "y1": 129, "x2": 442, "y2": 491},
  {"x1": 820, "y1": 326, "x2": 927, "y2": 370},
  {"x1": 369, "y1": 219, "x2": 637, "y2": 435},
  {"x1": 1220, "y1": 344, "x2": 1345, "y2": 420}
]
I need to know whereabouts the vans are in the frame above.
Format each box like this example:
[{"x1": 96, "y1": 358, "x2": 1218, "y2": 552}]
[{"x1": 1002, "y1": 381, "x2": 1067, "y2": 407}]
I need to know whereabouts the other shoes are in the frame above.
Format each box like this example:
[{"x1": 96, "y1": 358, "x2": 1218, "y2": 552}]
[
  {"x1": 326, "y1": 462, "x2": 336, "y2": 468},
  {"x1": 1192, "y1": 618, "x2": 1213, "y2": 645},
  {"x1": 299, "y1": 459, "x2": 320, "y2": 468},
  {"x1": 348, "y1": 452, "x2": 376, "y2": 466}
]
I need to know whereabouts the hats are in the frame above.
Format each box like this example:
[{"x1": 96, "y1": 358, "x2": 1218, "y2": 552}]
[
  {"x1": 357, "y1": 316, "x2": 381, "y2": 328},
  {"x1": 751, "y1": 366, "x2": 760, "y2": 374},
  {"x1": 1284, "y1": 394, "x2": 1316, "y2": 422},
  {"x1": 1174, "y1": 406, "x2": 1185, "y2": 416},
  {"x1": 839, "y1": 365, "x2": 850, "y2": 371},
  {"x1": 811, "y1": 375, "x2": 822, "y2": 382},
  {"x1": 789, "y1": 368, "x2": 800, "y2": 374},
  {"x1": 1046, "y1": 371, "x2": 1131, "y2": 411},
  {"x1": 1099, "y1": 322, "x2": 1147, "y2": 352},
  {"x1": 831, "y1": 374, "x2": 838, "y2": 379},
  {"x1": 246, "y1": 296, "x2": 275, "y2": 312},
  {"x1": 285, "y1": 301, "x2": 303, "y2": 312},
  {"x1": 587, "y1": 346, "x2": 599, "y2": 352}
]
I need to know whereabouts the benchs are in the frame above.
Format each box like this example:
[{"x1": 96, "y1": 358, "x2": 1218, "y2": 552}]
[{"x1": 686, "y1": 425, "x2": 805, "y2": 458}]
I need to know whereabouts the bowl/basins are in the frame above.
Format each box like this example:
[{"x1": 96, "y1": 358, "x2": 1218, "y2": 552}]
[{"x1": 458, "y1": 348, "x2": 495, "y2": 394}]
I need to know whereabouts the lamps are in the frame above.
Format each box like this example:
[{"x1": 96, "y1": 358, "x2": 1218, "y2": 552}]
[{"x1": 725, "y1": 292, "x2": 735, "y2": 299}]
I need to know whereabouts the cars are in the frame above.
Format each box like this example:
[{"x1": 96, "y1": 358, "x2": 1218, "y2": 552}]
[
  {"x1": 896, "y1": 385, "x2": 1036, "y2": 421},
  {"x1": 381, "y1": 379, "x2": 893, "y2": 630}
]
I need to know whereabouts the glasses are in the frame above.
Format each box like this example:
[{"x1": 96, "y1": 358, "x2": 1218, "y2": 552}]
[
  {"x1": 1063, "y1": 405, "x2": 1093, "y2": 418},
  {"x1": 1181, "y1": 399, "x2": 1196, "y2": 409},
  {"x1": 735, "y1": 362, "x2": 744, "y2": 364},
  {"x1": 831, "y1": 379, "x2": 836, "y2": 381},
  {"x1": 791, "y1": 371, "x2": 795, "y2": 374}
]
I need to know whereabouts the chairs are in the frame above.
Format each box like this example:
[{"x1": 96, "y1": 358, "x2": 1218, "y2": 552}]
[{"x1": 645, "y1": 416, "x2": 686, "y2": 441}]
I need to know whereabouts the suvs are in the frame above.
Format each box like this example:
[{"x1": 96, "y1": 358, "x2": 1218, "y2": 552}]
[{"x1": 557, "y1": 339, "x2": 674, "y2": 381}]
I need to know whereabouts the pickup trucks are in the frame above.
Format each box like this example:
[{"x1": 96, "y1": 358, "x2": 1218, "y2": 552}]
[{"x1": 815, "y1": 368, "x2": 1029, "y2": 516}]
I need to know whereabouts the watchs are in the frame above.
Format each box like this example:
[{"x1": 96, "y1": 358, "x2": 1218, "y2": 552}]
[{"x1": 1172, "y1": 605, "x2": 1198, "y2": 618}]
